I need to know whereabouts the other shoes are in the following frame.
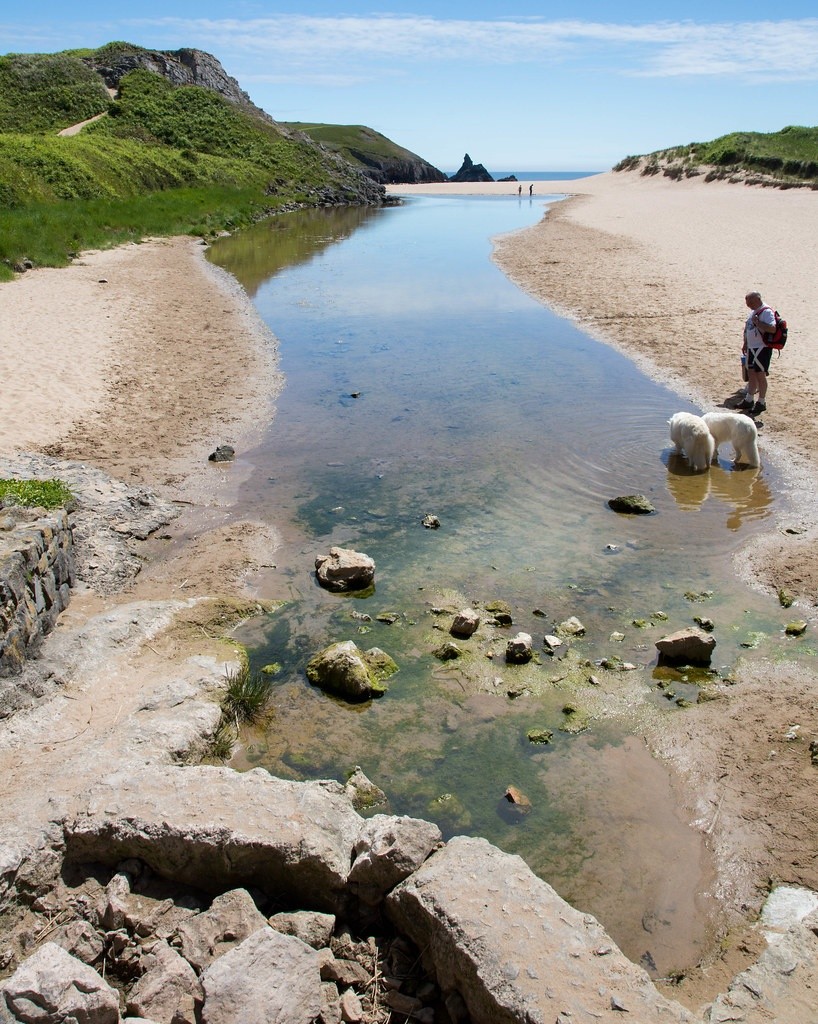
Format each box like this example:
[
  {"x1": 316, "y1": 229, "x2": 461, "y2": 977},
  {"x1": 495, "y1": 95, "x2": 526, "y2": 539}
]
[
  {"x1": 747, "y1": 401, "x2": 767, "y2": 414},
  {"x1": 733, "y1": 399, "x2": 754, "y2": 409}
]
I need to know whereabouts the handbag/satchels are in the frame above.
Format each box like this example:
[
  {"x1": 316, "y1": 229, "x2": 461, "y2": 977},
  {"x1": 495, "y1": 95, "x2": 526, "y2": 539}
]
[{"x1": 741, "y1": 354, "x2": 770, "y2": 382}]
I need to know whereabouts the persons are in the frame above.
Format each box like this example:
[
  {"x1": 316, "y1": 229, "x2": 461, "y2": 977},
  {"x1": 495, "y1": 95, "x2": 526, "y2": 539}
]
[
  {"x1": 733, "y1": 291, "x2": 777, "y2": 416},
  {"x1": 519, "y1": 185, "x2": 522, "y2": 195},
  {"x1": 529, "y1": 184, "x2": 534, "y2": 195}
]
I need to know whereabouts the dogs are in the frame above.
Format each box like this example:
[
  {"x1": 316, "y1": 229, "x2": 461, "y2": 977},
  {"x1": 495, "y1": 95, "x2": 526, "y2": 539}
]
[
  {"x1": 666, "y1": 411, "x2": 714, "y2": 474},
  {"x1": 699, "y1": 411, "x2": 761, "y2": 468}
]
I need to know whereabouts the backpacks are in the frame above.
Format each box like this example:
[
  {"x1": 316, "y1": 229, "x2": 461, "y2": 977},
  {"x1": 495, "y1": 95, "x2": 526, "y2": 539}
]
[{"x1": 753, "y1": 306, "x2": 787, "y2": 351}]
